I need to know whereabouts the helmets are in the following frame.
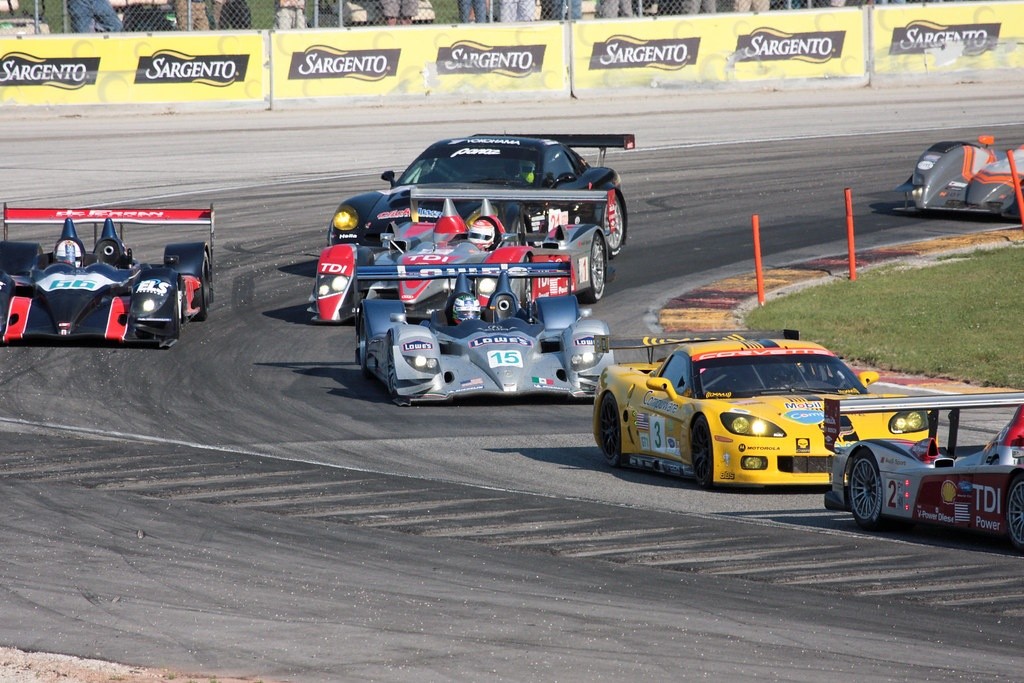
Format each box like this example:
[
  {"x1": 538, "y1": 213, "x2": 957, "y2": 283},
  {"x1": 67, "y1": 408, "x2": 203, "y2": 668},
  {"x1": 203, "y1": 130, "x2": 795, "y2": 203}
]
[
  {"x1": 467, "y1": 219, "x2": 495, "y2": 249},
  {"x1": 53, "y1": 240, "x2": 81, "y2": 268},
  {"x1": 763, "y1": 357, "x2": 795, "y2": 387},
  {"x1": 520, "y1": 159, "x2": 535, "y2": 178},
  {"x1": 452, "y1": 294, "x2": 481, "y2": 324}
]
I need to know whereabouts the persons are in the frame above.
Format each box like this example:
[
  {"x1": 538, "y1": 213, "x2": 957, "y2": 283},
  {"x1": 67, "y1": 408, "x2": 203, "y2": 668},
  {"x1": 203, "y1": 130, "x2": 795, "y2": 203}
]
[
  {"x1": 54, "y1": 240, "x2": 83, "y2": 269},
  {"x1": 64, "y1": 0, "x2": 843, "y2": 36},
  {"x1": 453, "y1": 294, "x2": 481, "y2": 324},
  {"x1": 467, "y1": 220, "x2": 495, "y2": 252},
  {"x1": 519, "y1": 158, "x2": 537, "y2": 183}
]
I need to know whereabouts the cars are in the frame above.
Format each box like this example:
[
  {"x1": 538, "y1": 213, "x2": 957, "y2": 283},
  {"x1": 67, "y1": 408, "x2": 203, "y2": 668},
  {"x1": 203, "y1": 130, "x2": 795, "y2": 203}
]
[
  {"x1": 591, "y1": 330, "x2": 938, "y2": 490},
  {"x1": 823, "y1": 390, "x2": 1024, "y2": 551},
  {"x1": 0, "y1": 201, "x2": 215, "y2": 347},
  {"x1": 892, "y1": 135, "x2": 1024, "y2": 219},
  {"x1": 352, "y1": 250, "x2": 613, "y2": 405},
  {"x1": 329, "y1": 134, "x2": 635, "y2": 256},
  {"x1": 307, "y1": 183, "x2": 616, "y2": 326}
]
